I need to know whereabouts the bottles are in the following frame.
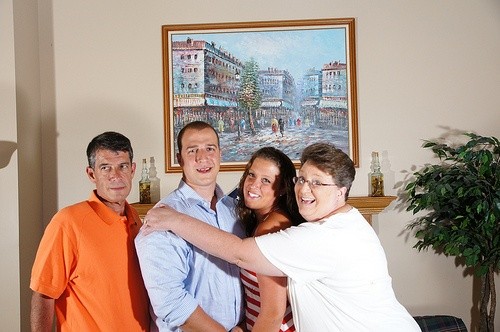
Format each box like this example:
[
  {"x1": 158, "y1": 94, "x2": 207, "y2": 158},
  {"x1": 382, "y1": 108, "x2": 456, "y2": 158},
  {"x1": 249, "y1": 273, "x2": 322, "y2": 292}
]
[
  {"x1": 139, "y1": 159, "x2": 151, "y2": 204},
  {"x1": 370, "y1": 152, "x2": 384, "y2": 197}
]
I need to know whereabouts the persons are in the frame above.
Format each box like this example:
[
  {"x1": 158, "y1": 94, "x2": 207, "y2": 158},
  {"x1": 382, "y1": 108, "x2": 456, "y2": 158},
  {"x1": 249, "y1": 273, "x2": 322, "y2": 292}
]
[
  {"x1": 143, "y1": 142, "x2": 423, "y2": 332},
  {"x1": 29, "y1": 131, "x2": 151, "y2": 332},
  {"x1": 229, "y1": 146, "x2": 303, "y2": 332},
  {"x1": 134, "y1": 120, "x2": 248, "y2": 332}
]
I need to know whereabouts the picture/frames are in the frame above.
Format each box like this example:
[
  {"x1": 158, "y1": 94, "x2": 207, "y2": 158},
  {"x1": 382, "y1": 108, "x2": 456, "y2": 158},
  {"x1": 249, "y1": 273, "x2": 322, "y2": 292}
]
[{"x1": 162, "y1": 17, "x2": 359, "y2": 173}]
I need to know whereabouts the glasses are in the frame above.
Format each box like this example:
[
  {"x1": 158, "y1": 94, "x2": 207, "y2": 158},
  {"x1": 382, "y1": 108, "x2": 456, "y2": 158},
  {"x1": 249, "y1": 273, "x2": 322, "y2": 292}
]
[{"x1": 292, "y1": 176, "x2": 337, "y2": 189}]
